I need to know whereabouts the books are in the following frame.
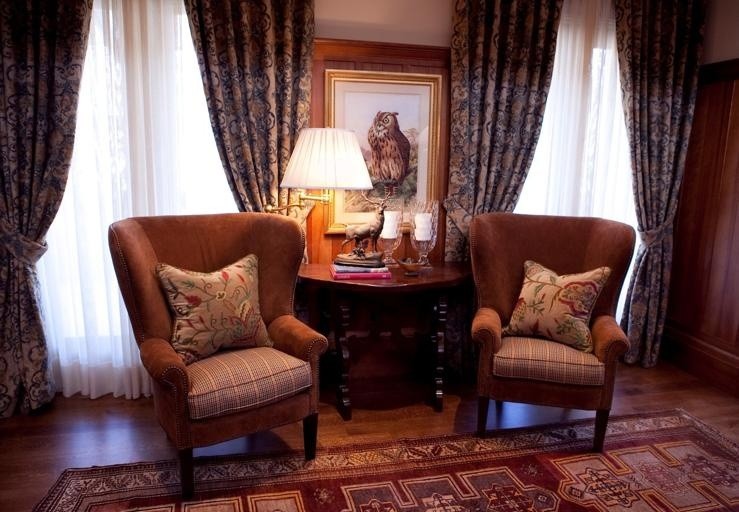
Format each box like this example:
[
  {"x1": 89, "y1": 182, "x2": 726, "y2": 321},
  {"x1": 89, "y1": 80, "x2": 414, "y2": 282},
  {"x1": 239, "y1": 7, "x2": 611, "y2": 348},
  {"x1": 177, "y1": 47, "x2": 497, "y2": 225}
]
[{"x1": 328, "y1": 264, "x2": 391, "y2": 280}]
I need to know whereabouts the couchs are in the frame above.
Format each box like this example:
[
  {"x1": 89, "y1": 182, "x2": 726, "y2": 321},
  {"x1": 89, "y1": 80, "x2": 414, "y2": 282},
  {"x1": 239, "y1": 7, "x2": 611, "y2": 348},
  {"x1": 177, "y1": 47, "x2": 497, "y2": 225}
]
[
  {"x1": 108, "y1": 212, "x2": 329, "y2": 498},
  {"x1": 469, "y1": 212, "x2": 636, "y2": 451}
]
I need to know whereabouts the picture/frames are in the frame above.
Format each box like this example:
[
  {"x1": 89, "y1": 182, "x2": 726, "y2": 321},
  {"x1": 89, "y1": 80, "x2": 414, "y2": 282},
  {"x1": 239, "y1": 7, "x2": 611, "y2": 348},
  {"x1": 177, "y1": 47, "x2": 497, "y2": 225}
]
[{"x1": 323, "y1": 69, "x2": 443, "y2": 234}]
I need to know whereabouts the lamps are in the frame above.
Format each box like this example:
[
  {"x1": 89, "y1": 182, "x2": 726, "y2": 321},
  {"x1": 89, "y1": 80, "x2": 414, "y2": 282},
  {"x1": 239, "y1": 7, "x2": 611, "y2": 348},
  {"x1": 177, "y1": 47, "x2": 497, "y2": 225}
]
[{"x1": 265, "y1": 127, "x2": 374, "y2": 211}]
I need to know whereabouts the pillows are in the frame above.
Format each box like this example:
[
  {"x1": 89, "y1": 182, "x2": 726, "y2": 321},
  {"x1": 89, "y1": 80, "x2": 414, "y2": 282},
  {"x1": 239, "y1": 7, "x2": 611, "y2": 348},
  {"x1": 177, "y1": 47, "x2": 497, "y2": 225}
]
[
  {"x1": 502, "y1": 258, "x2": 612, "y2": 352},
  {"x1": 156, "y1": 254, "x2": 274, "y2": 364}
]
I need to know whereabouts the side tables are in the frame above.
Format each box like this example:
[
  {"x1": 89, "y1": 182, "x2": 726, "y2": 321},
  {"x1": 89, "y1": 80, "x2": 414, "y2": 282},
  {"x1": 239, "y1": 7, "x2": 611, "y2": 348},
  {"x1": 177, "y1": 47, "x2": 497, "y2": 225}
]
[{"x1": 297, "y1": 264, "x2": 472, "y2": 420}]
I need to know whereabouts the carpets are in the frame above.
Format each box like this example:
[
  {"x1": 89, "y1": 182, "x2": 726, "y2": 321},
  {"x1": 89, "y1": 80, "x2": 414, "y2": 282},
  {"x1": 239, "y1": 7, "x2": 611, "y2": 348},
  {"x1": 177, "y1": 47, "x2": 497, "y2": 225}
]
[{"x1": 32, "y1": 406, "x2": 739, "y2": 511}]
